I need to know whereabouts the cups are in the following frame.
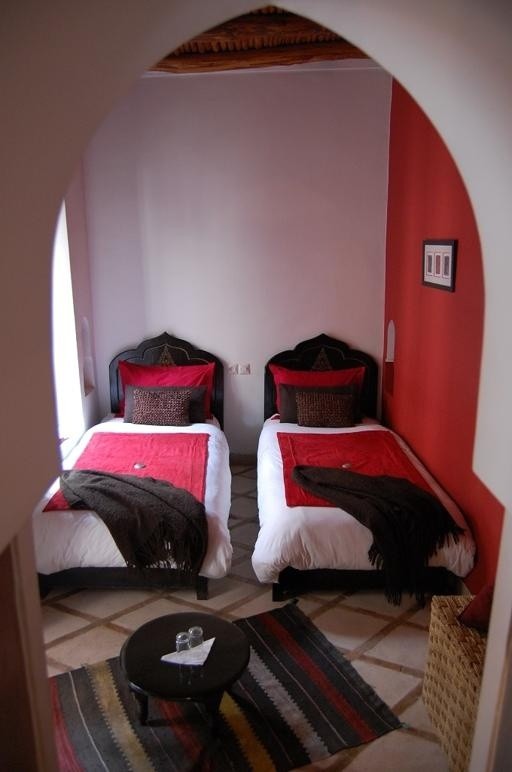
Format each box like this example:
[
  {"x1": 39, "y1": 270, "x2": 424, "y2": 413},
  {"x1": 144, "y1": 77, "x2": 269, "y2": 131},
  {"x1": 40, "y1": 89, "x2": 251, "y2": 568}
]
[
  {"x1": 175, "y1": 631, "x2": 192, "y2": 664},
  {"x1": 189, "y1": 627, "x2": 208, "y2": 663}
]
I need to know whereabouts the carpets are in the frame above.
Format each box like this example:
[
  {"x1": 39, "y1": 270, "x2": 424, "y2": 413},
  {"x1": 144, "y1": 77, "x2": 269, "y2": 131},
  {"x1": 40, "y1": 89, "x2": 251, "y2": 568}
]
[{"x1": 47, "y1": 595, "x2": 412, "y2": 772}]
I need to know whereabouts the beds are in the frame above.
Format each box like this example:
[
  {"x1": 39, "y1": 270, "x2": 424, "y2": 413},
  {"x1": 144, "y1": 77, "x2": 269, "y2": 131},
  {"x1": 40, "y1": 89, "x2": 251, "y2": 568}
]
[
  {"x1": 23, "y1": 330, "x2": 234, "y2": 602},
  {"x1": 249, "y1": 333, "x2": 477, "y2": 607}
]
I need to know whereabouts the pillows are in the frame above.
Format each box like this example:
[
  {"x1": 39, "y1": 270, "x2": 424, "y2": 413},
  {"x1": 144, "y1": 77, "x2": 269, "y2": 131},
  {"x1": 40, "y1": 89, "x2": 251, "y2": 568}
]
[
  {"x1": 117, "y1": 351, "x2": 221, "y2": 429},
  {"x1": 263, "y1": 357, "x2": 378, "y2": 429},
  {"x1": 458, "y1": 583, "x2": 493, "y2": 635}
]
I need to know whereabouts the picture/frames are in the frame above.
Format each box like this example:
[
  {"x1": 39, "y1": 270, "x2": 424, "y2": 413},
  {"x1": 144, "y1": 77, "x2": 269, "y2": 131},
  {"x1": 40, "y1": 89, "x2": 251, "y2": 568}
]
[{"x1": 419, "y1": 238, "x2": 462, "y2": 293}]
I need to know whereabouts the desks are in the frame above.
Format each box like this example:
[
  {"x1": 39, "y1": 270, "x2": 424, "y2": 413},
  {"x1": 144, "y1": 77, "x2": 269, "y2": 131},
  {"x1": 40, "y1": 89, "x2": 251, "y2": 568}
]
[{"x1": 116, "y1": 613, "x2": 259, "y2": 746}]
